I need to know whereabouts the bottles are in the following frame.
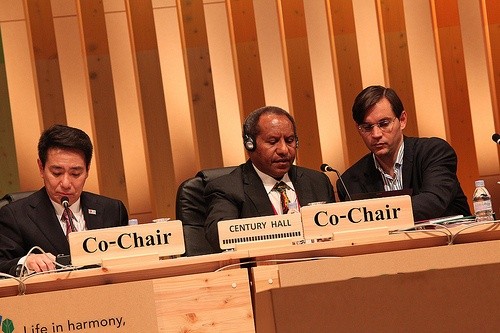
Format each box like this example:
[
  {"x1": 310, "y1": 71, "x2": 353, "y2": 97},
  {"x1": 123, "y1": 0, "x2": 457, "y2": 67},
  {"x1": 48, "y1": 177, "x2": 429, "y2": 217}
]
[
  {"x1": 129, "y1": 218, "x2": 138, "y2": 226},
  {"x1": 287, "y1": 202, "x2": 304, "y2": 245},
  {"x1": 472, "y1": 179, "x2": 494, "y2": 222}
]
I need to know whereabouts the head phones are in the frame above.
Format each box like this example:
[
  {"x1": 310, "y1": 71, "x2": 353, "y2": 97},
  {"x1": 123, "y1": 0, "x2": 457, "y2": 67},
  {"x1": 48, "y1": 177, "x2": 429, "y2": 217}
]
[{"x1": 242, "y1": 112, "x2": 299, "y2": 152}]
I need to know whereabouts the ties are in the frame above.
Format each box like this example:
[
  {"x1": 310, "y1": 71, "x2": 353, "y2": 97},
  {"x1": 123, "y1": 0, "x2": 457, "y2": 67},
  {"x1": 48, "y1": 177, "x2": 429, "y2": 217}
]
[
  {"x1": 62, "y1": 208, "x2": 78, "y2": 238},
  {"x1": 275, "y1": 182, "x2": 292, "y2": 214}
]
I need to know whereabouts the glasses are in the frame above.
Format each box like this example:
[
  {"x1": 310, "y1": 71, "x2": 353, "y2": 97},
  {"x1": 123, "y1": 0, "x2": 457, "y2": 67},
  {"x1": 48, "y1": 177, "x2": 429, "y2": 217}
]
[{"x1": 357, "y1": 116, "x2": 396, "y2": 134}]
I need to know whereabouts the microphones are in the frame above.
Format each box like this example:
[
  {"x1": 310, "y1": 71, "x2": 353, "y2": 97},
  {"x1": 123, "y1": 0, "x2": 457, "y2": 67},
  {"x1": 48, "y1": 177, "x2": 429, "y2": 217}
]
[
  {"x1": 492, "y1": 134, "x2": 500, "y2": 144},
  {"x1": 61, "y1": 196, "x2": 74, "y2": 233},
  {"x1": 320, "y1": 163, "x2": 352, "y2": 201}
]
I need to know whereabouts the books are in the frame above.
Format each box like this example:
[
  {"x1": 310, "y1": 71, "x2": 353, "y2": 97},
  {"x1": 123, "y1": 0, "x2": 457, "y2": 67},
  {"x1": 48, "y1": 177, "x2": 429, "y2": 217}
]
[{"x1": 414, "y1": 215, "x2": 463, "y2": 224}]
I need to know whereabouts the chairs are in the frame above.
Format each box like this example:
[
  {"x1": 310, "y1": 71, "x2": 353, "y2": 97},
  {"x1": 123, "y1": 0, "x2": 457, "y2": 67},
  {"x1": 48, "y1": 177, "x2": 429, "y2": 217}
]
[
  {"x1": 176, "y1": 166, "x2": 238, "y2": 257},
  {"x1": 0, "y1": 191, "x2": 36, "y2": 210}
]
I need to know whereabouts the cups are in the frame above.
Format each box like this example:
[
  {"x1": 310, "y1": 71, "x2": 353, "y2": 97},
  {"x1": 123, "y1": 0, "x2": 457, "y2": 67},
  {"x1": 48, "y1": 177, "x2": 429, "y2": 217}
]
[
  {"x1": 308, "y1": 201, "x2": 330, "y2": 241},
  {"x1": 152, "y1": 217, "x2": 171, "y2": 260}
]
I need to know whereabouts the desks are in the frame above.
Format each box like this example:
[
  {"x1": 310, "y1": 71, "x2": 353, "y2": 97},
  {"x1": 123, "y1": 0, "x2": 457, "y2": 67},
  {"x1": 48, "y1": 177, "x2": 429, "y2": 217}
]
[{"x1": 0, "y1": 223, "x2": 500, "y2": 333}]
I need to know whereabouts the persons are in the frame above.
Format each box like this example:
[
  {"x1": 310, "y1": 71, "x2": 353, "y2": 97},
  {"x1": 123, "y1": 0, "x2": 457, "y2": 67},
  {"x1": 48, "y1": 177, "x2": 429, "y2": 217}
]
[
  {"x1": 335, "y1": 85, "x2": 473, "y2": 229},
  {"x1": 203, "y1": 106, "x2": 336, "y2": 252},
  {"x1": 0, "y1": 125, "x2": 129, "y2": 279}
]
